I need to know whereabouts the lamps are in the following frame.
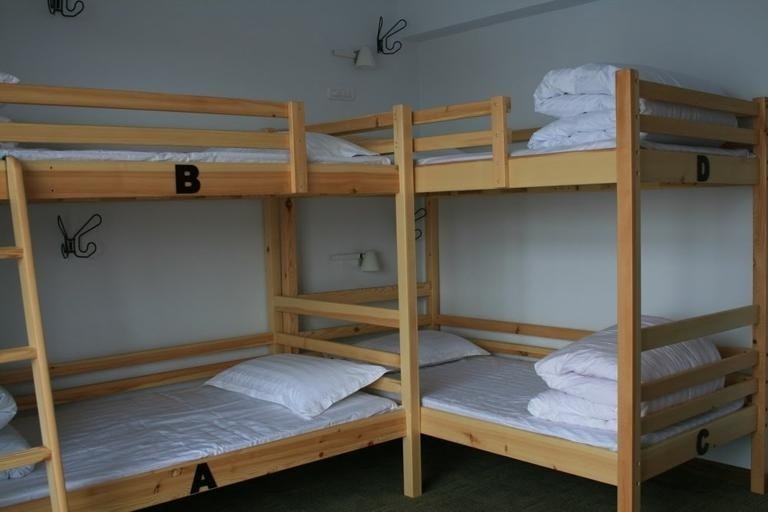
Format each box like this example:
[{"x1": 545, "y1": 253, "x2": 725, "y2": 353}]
[{"x1": 331, "y1": 46, "x2": 377, "y2": 70}]
